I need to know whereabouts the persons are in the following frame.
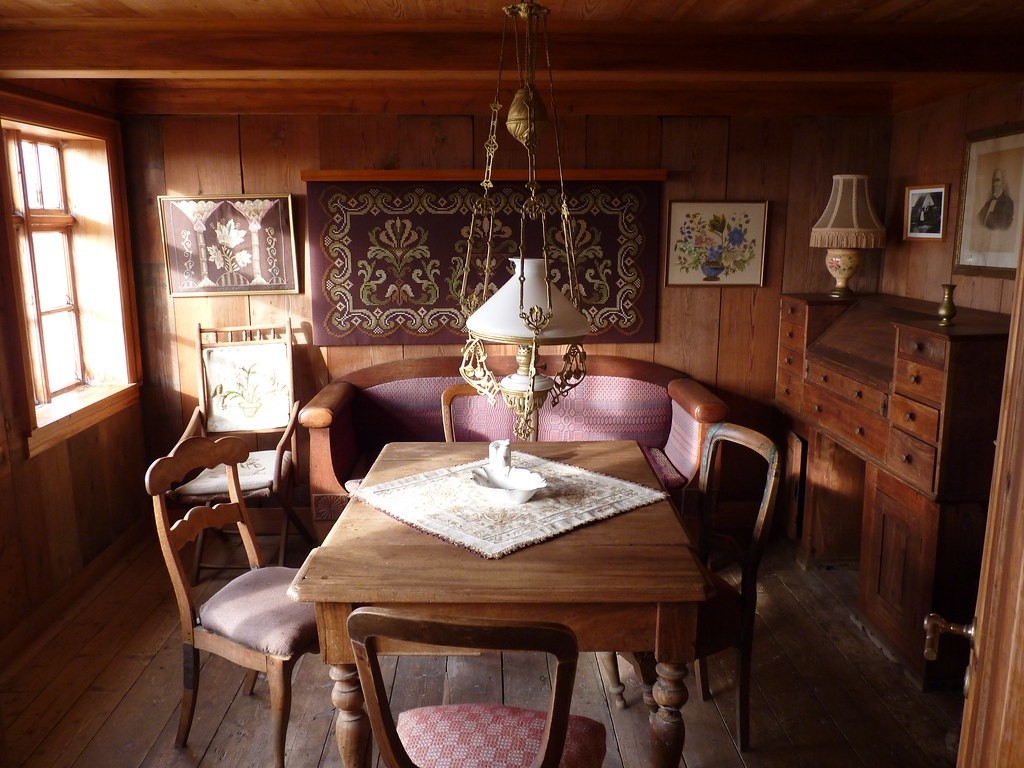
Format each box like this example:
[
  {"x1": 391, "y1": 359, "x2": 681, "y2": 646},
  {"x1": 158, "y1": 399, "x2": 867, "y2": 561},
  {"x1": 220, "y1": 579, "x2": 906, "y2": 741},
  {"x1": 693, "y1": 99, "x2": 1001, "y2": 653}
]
[{"x1": 913, "y1": 202, "x2": 939, "y2": 223}]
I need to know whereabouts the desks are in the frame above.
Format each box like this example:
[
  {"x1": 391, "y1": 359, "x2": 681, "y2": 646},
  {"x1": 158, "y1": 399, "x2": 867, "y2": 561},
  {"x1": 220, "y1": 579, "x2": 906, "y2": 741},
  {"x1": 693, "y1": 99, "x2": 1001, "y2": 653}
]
[{"x1": 283, "y1": 434, "x2": 709, "y2": 768}]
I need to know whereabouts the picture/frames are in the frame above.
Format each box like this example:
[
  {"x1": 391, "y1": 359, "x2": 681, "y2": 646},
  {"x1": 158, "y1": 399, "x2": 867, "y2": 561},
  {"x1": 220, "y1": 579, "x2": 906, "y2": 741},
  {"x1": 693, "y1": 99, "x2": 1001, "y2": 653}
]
[
  {"x1": 949, "y1": 117, "x2": 1024, "y2": 282},
  {"x1": 664, "y1": 196, "x2": 773, "y2": 291},
  {"x1": 156, "y1": 192, "x2": 304, "y2": 300},
  {"x1": 899, "y1": 182, "x2": 946, "y2": 243}
]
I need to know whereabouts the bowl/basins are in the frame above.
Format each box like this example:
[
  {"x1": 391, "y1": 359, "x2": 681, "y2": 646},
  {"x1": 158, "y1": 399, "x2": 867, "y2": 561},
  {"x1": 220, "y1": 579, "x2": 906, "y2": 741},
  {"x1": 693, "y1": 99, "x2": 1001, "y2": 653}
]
[{"x1": 469, "y1": 465, "x2": 548, "y2": 504}]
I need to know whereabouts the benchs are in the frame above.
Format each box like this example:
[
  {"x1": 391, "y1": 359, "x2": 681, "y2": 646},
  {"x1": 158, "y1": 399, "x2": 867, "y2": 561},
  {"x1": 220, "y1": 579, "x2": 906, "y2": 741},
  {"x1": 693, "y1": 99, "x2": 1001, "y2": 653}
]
[{"x1": 296, "y1": 352, "x2": 737, "y2": 555}]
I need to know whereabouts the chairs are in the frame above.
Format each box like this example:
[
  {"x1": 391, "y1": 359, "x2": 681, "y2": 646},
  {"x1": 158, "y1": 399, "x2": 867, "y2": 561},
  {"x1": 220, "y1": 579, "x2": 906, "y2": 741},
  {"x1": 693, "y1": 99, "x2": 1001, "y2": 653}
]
[
  {"x1": 164, "y1": 317, "x2": 310, "y2": 589},
  {"x1": 345, "y1": 601, "x2": 609, "y2": 768},
  {"x1": 632, "y1": 421, "x2": 786, "y2": 754},
  {"x1": 143, "y1": 433, "x2": 323, "y2": 768}
]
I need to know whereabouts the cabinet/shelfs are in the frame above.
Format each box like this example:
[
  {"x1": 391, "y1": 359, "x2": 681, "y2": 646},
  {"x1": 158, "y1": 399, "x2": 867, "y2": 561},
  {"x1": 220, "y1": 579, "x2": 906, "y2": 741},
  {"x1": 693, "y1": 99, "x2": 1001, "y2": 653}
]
[{"x1": 775, "y1": 290, "x2": 1012, "y2": 692}]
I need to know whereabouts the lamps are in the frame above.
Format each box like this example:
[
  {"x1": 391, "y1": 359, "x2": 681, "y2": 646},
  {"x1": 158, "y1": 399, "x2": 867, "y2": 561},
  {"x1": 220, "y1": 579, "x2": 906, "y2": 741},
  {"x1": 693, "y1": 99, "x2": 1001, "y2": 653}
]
[
  {"x1": 457, "y1": 0, "x2": 602, "y2": 443},
  {"x1": 805, "y1": 173, "x2": 886, "y2": 300}
]
